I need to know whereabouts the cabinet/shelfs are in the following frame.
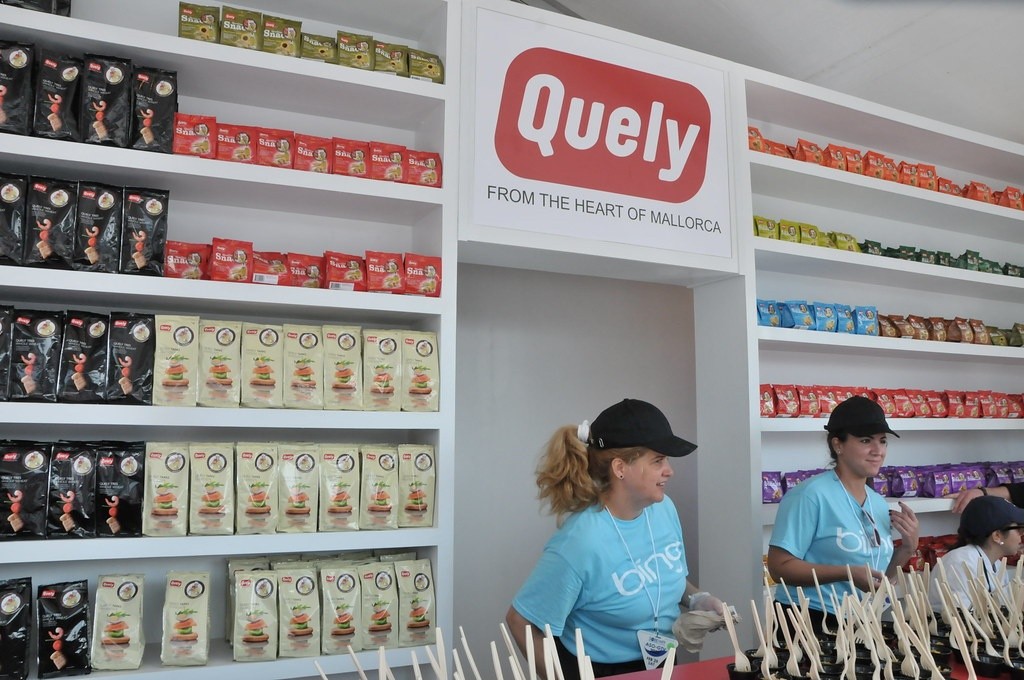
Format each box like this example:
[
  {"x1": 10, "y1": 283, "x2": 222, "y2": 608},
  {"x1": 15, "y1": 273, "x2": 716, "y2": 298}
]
[
  {"x1": 694, "y1": 65, "x2": 1024, "y2": 661},
  {"x1": 0, "y1": 0, "x2": 458, "y2": 680}
]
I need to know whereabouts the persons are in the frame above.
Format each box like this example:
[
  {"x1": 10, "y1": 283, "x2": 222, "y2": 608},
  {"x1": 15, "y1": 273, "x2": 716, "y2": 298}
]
[
  {"x1": 768, "y1": 395, "x2": 919, "y2": 642},
  {"x1": 945, "y1": 481, "x2": 1024, "y2": 514},
  {"x1": 928, "y1": 495, "x2": 1024, "y2": 614},
  {"x1": 507, "y1": 398, "x2": 723, "y2": 680}
]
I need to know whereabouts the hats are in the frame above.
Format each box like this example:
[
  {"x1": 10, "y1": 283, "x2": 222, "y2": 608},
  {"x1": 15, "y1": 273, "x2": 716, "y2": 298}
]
[
  {"x1": 824, "y1": 394, "x2": 902, "y2": 441},
  {"x1": 588, "y1": 397, "x2": 698, "y2": 456},
  {"x1": 957, "y1": 495, "x2": 1024, "y2": 535}
]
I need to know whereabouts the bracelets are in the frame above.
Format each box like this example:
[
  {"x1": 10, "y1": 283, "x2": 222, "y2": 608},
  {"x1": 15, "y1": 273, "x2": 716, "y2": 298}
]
[{"x1": 978, "y1": 487, "x2": 987, "y2": 496}]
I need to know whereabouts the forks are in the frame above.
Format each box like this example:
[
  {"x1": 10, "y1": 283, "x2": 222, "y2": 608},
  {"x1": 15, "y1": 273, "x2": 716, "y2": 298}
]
[{"x1": 720, "y1": 555, "x2": 1024, "y2": 680}]
